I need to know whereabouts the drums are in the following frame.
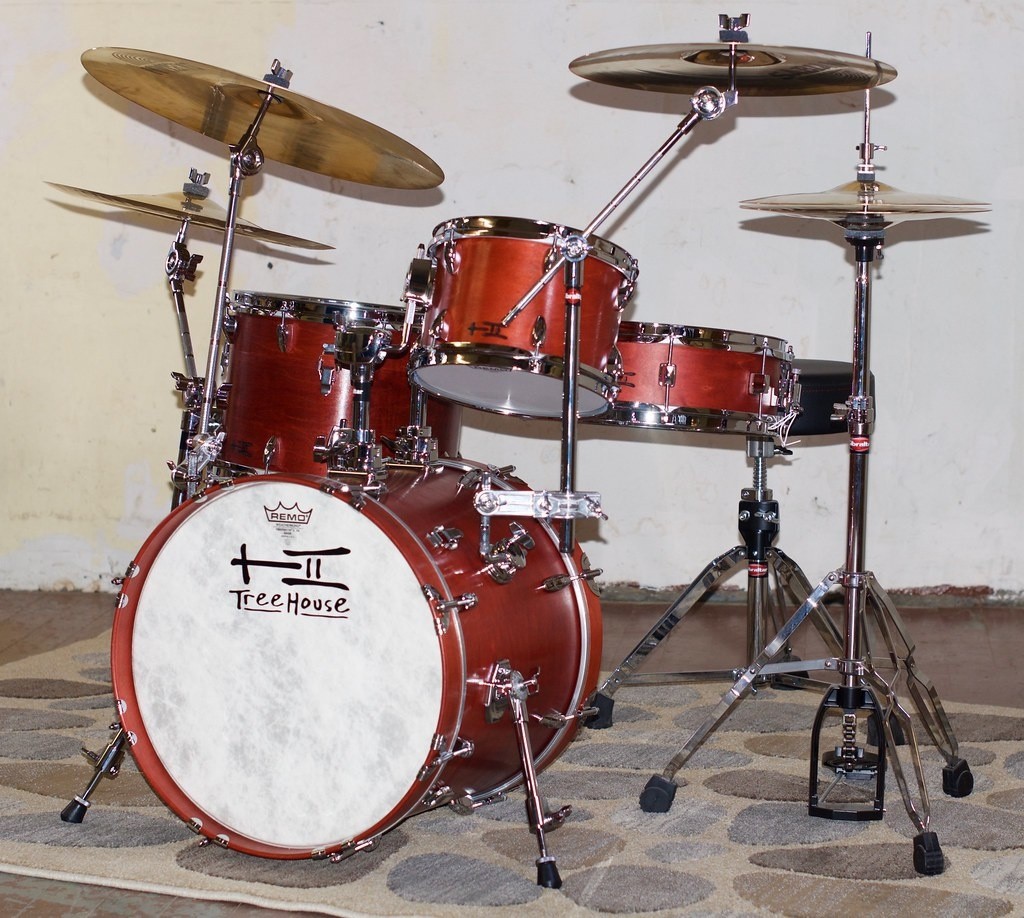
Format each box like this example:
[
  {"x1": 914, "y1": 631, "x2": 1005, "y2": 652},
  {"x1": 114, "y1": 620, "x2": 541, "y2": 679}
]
[
  {"x1": 583, "y1": 320, "x2": 801, "y2": 434},
  {"x1": 202, "y1": 290, "x2": 447, "y2": 474},
  {"x1": 408, "y1": 216, "x2": 640, "y2": 417},
  {"x1": 111, "y1": 451, "x2": 606, "y2": 860}
]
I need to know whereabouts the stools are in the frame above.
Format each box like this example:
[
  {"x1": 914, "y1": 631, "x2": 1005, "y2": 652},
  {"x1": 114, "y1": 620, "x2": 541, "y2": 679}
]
[{"x1": 582, "y1": 357, "x2": 908, "y2": 748}]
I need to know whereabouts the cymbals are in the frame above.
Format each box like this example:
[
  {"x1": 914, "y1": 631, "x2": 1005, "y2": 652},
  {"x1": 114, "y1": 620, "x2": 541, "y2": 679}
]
[
  {"x1": 46, "y1": 179, "x2": 338, "y2": 251},
  {"x1": 80, "y1": 45, "x2": 444, "y2": 189},
  {"x1": 740, "y1": 179, "x2": 994, "y2": 228},
  {"x1": 568, "y1": 42, "x2": 898, "y2": 97}
]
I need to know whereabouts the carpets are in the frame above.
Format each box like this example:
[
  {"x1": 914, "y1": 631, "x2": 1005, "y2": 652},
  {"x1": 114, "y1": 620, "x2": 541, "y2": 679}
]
[{"x1": 0, "y1": 629, "x2": 1024, "y2": 918}]
